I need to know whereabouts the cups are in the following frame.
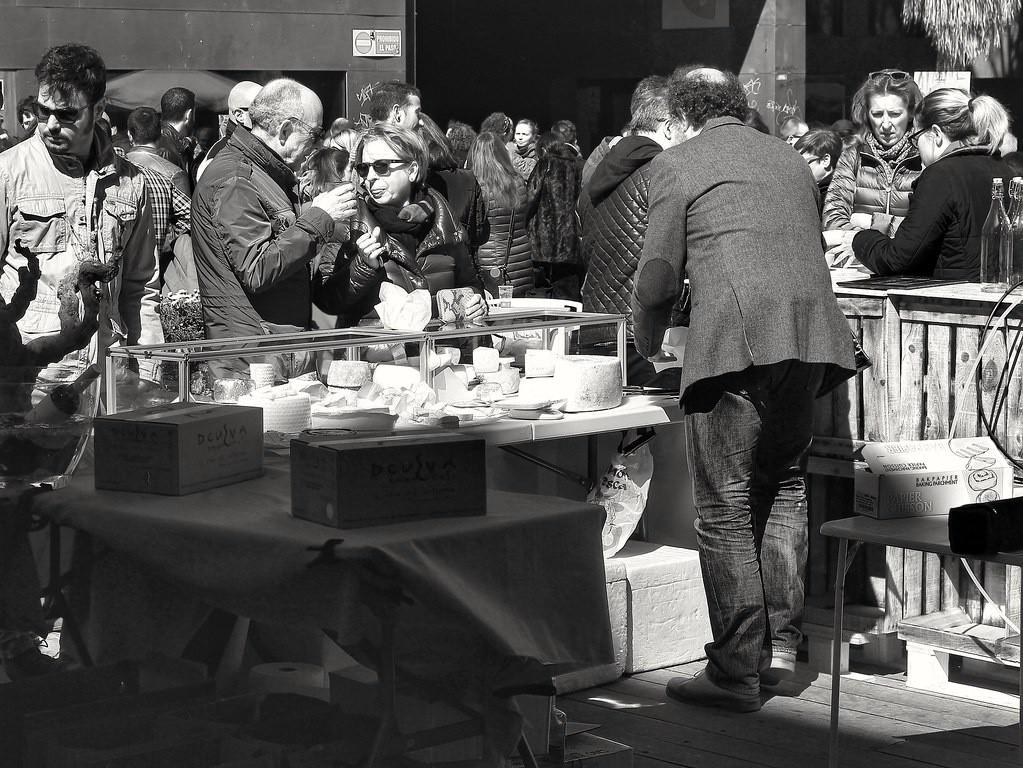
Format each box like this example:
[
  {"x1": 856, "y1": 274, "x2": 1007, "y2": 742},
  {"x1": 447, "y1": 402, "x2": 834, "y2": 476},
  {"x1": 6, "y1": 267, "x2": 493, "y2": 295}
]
[
  {"x1": 322, "y1": 181, "x2": 352, "y2": 212},
  {"x1": 214, "y1": 379, "x2": 246, "y2": 406},
  {"x1": 497, "y1": 285, "x2": 514, "y2": 309}
]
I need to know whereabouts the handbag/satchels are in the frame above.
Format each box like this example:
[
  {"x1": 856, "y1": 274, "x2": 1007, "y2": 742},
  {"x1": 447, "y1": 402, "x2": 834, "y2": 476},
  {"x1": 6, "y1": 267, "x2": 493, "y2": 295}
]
[
  {"x1": 480, "y1": 266, "x2": 508, "y2": 299},
  {"x1": 586, "y1": 430, "x2": 653, "y2": 559}
]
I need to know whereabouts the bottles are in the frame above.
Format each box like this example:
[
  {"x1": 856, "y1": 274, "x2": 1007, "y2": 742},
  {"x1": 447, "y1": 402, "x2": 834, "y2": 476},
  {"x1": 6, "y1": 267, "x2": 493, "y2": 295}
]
[
  {"x1": 979, "y1": 178, "x2": 1014, "y2": 295},
  {"x1": 999, "y1": 177, "x2": 1022, "y2": 293}
]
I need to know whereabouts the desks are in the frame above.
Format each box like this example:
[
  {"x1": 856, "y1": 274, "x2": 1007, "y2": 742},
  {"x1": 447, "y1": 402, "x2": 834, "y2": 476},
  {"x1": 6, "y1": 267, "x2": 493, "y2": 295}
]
[
  {"x1": 238, "y1": 391, "x2": 682, "y2": 545},
  {"x1": 820, "y1": 512, "x2": 1023, "y2": 768},
  {"x1": 0, "y1": 478, "x2": 619, "y2": 768}
]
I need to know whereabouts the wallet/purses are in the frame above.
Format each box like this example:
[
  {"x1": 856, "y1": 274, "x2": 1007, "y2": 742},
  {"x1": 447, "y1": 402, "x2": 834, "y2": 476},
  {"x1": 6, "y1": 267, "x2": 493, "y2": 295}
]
[{"x1": 436, "y1": 287, "x2": 476, "y2": 323}]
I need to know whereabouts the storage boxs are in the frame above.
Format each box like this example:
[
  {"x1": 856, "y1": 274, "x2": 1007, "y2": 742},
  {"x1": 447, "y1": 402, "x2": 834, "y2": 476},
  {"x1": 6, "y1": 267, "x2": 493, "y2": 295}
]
[
  {"x1": 92, "y1": 401, "x2": 269, "y2": 494},
  {"x1": 852, "y1": 433, "x2": 1014, "y2": 523},
  {"x1": 289, "y1": 426, "x2": 486, "y2": 529}
]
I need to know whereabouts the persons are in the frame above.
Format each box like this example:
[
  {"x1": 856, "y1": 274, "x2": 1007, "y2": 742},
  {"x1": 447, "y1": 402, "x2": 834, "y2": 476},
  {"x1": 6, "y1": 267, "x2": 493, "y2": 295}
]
[
  {"x1": 632, "y1": 64, "x2": 874, "y2": 713},
  {"x1": 743, "y1": 68, "x2": 1023, "y2": 282},
  {"x1": 0, "y1": 44, "x2": 166, "y2": 677},
  {"x1": 0, "y1": 78, "x2": 691, "y2": 386}
]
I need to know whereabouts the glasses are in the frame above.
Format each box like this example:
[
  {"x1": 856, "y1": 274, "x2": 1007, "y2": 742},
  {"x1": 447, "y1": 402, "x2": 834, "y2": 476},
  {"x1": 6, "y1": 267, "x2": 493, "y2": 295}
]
[
  {"x1": 31, "y1": 97, "x2": 98, "y2": 125},
  {"x1": 869, "y1": 71, "x2": 908, "y2": 82},
  {"x1": 353, "y1": 159, "x2": 410, "y2": 179},
  {"x1": 286, "y1": 117, "x2": 324, "y2": 143},
  {"x1": 907, "y1": 124, "x2": 944, "y2": 149}
]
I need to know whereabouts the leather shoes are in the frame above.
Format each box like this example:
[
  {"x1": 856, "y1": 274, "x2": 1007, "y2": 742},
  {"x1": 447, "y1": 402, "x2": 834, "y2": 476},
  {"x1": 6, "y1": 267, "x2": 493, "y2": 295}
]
[
  {"x1": 759, "y1": 647, "x2": 796, "y2": 686},
  {"x1": 665, "y1": 668, "x2": 760, "y2": 713}
]
